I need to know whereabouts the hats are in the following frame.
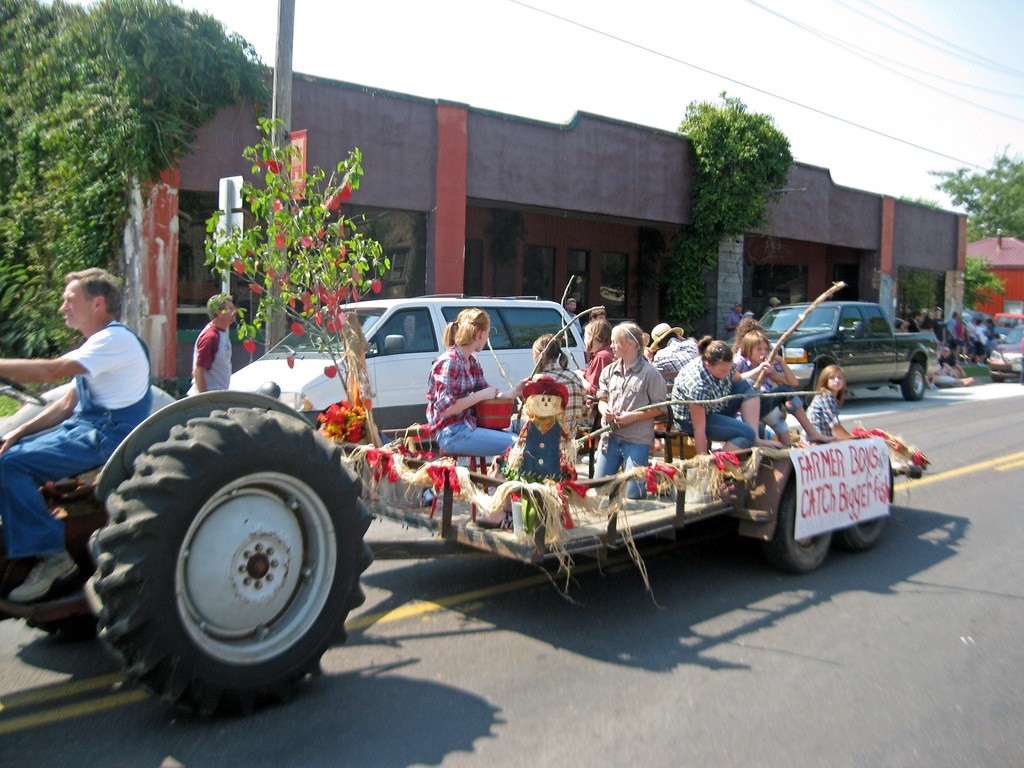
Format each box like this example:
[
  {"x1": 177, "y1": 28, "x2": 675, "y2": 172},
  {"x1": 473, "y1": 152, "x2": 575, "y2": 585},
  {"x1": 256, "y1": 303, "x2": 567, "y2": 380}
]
[
  {"x1": 768, "y1": 297, "x2": 780, "y2": 305},
  {"x1": 743, "y1": 311, "x2": 754, "y2": 317},
  {"x1": 735, "y1": 302, "x2": 742, "y2": 307},
  {"x1": 567, "y1": 298, "x2": 576, "y2": 305},
  {"x1": 649, "y1": 322, "x2": 684, "y2": 353}
]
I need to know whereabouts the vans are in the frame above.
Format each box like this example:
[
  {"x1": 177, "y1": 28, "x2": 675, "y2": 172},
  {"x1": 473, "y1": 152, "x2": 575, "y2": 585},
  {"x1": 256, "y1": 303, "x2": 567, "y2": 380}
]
[{"x1": 232, "y1": 293, "x2": 588, "y2": 450}]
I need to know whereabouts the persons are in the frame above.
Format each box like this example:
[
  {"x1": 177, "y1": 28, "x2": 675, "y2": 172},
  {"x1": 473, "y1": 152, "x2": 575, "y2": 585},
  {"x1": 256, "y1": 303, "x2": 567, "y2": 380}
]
[
  {"x1": 566, "y1": 298, "x2": 582, "y2": 337},
  {"x1": 0, "y1": 267, "x2": 154, "y2": 603},
  {"x1": 743, "y1": 309, "x2": 754, "y2": 318},
  {"x1": 724, "y1": 302, "x2": 743, "y2": 339},
  {"x1": 900, "y1": 305, "x2": 1008, "y2": 388},
  {"x1": 425, "y1": 308, "x2": 519, "y2": 456},
  {"x1": 508, "y1": 318, "x2": 853, "y2": 499},
  {"x1": 763, "y1": 297, "x2": 781, "y2": 316},
  {"x1": 186, "y1": 292, "x2": 237, "y2": 396},
  {"x1": 591, "y1": 308, "x2": 606, "y2": 319},
  {"x1": 509, "y1": 374, "x2": 571, "y2": 482}
]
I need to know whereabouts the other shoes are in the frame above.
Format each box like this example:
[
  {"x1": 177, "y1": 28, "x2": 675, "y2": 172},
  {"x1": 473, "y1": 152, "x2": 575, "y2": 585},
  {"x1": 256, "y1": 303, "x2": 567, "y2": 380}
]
[
  {"x1": 581, "y1": 450, "x2": 598, "y2": 465},
  {"x1": 955, "y1": 357, "x2": 987, "y2": 369},
  {"x1": 476, "y1": 515, "x2": 511, "y2": 528},
  {"x1": 964, "y1": 377, "x2": 973, "y2": 386},
  {"x1": 723, "y1": 476, "x2": 739, "y2": 493},
  {"x1": 931, "y1": 384, "x2": 938, "y2": 390},
  {"x1": 927, "y1": 384, "x2": 934, "y2": 391}
]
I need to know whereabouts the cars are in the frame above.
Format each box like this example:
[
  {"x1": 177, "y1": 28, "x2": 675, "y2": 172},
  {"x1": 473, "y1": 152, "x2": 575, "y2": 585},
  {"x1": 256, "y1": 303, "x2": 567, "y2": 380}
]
[{"x1": 986, "y1": 326, "x2": 1024, "y2": 382}]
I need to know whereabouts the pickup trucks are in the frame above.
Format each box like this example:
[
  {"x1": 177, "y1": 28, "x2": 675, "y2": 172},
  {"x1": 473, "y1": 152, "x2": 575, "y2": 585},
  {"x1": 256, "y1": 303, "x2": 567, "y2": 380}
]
[{"x1": 759, "y1": 301, "x2": 942, "y2": 402}]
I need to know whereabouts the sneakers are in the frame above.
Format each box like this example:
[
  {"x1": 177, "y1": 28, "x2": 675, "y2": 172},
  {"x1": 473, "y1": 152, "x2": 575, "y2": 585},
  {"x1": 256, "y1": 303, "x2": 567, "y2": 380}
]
[{"x1": 9, "y1": 552, "x2": 76, "y2": 602}]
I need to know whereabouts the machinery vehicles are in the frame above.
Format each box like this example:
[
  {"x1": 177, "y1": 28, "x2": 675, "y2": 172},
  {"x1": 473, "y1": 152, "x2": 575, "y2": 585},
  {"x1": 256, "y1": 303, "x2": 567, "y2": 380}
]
[{"x1": 1, "y1": 376, "x2": 374, "y2": 717}]
